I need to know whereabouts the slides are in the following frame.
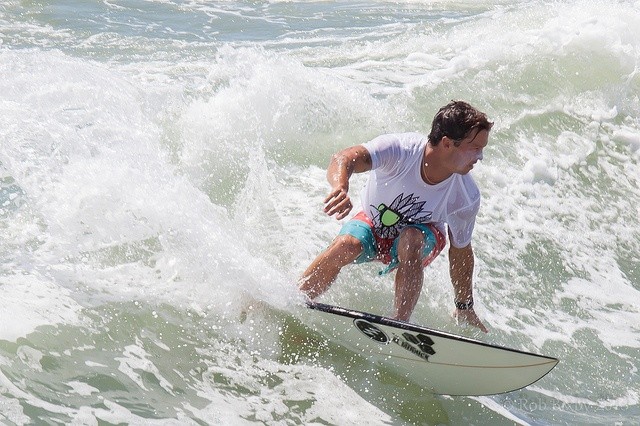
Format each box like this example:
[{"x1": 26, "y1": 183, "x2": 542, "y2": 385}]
[{"x1": 298, "y1": 299, "x2": 560, "y2": 396}]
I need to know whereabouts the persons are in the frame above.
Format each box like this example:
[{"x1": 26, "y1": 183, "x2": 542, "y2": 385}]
[{"x1": 294, "y1": 100, "x2": 494, "y2": 333}]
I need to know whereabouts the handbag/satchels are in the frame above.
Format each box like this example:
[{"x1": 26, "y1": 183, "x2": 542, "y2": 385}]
[{"x1": 454, "y1": 296, "x2": 474, "y2": 310}]
